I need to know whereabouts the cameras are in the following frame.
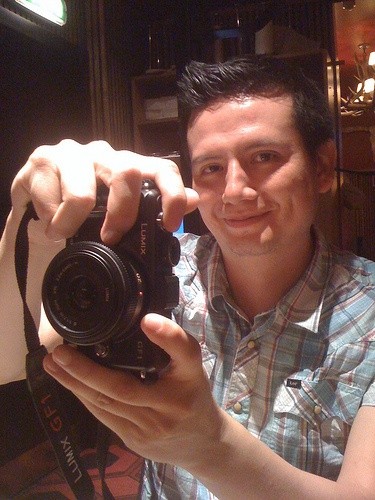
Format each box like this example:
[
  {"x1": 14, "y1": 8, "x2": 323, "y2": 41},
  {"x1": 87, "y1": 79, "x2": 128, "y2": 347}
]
[{"x1": 41, "y1": 179, "x2": 181, "y2": 381}]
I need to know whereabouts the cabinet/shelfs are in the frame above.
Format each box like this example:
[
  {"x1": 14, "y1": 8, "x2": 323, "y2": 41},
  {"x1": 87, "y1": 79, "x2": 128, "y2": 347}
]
[{"x1": 131, "y1": 50, "x2": 342, "y2": 249}]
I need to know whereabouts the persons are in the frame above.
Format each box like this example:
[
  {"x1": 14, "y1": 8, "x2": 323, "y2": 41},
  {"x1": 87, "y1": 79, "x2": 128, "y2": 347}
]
[{"x1": 1, "y1": 54, "x2": 375, "y2": 500}]
